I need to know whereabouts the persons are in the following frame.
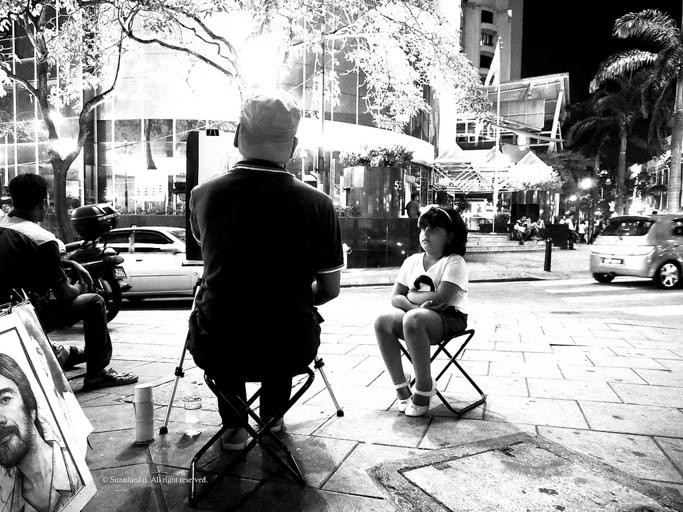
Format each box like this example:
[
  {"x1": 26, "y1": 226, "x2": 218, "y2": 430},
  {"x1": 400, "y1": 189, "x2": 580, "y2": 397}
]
[
  {"x1": 188, "y1": 90, "x2": 345, "y2": 450},
  {"x1": 374, "y1": 207, "x2": 469, "y2": 416},
  {"x1": 514, "y1": 213, "x2": 606, "y2": 250},
  {"x1": 0, "y1": 174, "x2": 138, "y2": 389},
  {"x1": 406, "y1": 193, "x2": 422, "y2": 253},
  {"x1": 0, "y1": 354, "x2": 83, "y2": 512}
]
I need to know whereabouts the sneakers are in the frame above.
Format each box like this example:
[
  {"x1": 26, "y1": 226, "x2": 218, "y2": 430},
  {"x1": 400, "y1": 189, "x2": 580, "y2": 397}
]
[
  {"x1": 83, "y1": 368, "x2": 138, "y2": 389},
  {"x1": 60, "y1": 346, "x2": 85, "y2": 369},
  {"x1": 260, "y1": 413, "x2": 284, "y2": 433},
  {"x1": 222, "y1": 427, "x2": 248, "y2": 450}
]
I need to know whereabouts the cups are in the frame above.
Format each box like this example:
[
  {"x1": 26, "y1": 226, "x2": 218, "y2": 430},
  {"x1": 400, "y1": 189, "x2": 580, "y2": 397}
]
[{"x1": 132, "y1": 383, "x2": 155, "y2": 446}]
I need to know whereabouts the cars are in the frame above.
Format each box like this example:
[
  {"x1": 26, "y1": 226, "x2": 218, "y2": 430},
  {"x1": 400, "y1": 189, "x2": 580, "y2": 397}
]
[
  {"x1": 64, "y1": 226, "x2": 204, "y2": 298},
  {"x1": 589, "y1": 214, "x2": 683, "y2": 290}
]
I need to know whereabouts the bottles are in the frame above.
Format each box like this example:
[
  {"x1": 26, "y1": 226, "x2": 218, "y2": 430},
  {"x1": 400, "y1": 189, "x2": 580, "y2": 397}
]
[{"x1": 185, "y1": 380, "x2": 203, "y2": 439}]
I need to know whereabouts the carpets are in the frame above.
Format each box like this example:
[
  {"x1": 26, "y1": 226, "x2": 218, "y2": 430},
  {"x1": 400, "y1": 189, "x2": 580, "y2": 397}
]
[{"x1": 369, "y1": 432, "x2": 682, "y2": 511}]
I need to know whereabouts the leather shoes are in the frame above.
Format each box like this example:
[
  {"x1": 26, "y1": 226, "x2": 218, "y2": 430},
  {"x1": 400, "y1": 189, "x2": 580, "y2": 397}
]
[{"x1": 393, "y1": 374, "x2": 435, "y2": 418}]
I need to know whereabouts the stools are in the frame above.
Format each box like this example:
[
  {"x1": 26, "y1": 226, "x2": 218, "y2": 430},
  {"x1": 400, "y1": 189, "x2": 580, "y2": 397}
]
[{"x1": 393, "y1": 328, "x2": 485, "y2": 415}]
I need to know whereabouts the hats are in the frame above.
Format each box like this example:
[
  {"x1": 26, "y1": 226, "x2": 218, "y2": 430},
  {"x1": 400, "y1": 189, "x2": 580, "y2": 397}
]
[{"x1": 238, "y1": 88, "x2": 300, "y2": 164}]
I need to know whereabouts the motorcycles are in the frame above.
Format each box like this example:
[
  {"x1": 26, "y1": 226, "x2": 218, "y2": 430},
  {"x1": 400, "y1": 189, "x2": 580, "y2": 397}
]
[{"x1": 52, "y1": 203, "x2": 127, "y2": 324}]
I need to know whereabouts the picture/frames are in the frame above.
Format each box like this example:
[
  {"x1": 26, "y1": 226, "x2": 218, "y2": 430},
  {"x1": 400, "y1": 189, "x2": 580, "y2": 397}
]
[{"x1": 0, "y1": 311, "x2": 97, "y2": 512}]
